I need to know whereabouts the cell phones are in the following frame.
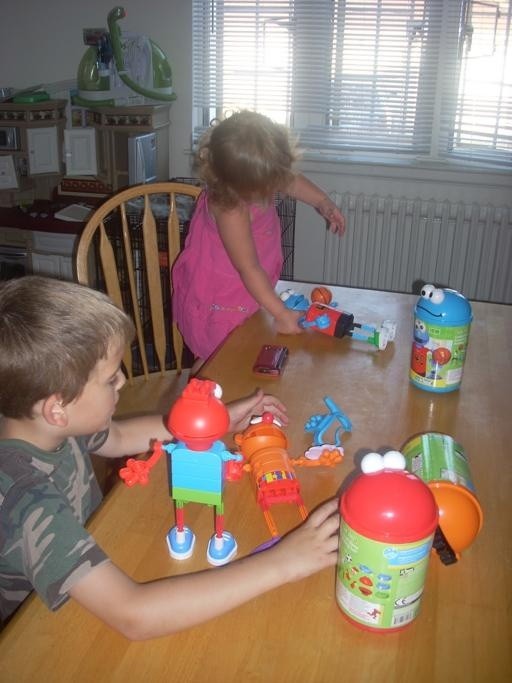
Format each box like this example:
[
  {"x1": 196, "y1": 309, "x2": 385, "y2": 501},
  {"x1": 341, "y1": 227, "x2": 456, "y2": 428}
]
[{"x1": 254, "y1": 344, "x2": 288, "y2": 376}]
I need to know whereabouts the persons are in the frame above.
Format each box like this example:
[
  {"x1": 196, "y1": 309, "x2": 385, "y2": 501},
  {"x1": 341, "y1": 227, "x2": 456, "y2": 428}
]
[
  {"x1": 223, "y1": 410, "x2": 343, "y2": 557},
  {"x1": 1, "y1": 273, "x2": 343, "y2": 643},
  {"x1": 170, "y1": 108, "x2": 348, "y2": 357},
  {"x1": 276, "y1": 285, "x2": 397, "y2": 352},
  {"x1": 116, "y1": 378, "x2": 245, "y2": 565}
]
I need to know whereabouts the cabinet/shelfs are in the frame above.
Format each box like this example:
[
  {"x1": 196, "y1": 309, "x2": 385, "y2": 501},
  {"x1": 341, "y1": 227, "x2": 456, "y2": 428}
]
[{"x1": 0, "y1": 95, "x2": 172, "y2": 288}]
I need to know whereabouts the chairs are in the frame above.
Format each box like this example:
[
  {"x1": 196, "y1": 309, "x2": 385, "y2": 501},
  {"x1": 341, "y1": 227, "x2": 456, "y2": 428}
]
[{"x1": 76, "y1": 182, "x2": 206, "y2": 497}]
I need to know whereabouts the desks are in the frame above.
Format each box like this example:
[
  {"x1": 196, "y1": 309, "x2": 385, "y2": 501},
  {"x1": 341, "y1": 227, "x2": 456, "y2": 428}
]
[{"x1": 0, "y1": 278, "x2": 512, "y2": 682}]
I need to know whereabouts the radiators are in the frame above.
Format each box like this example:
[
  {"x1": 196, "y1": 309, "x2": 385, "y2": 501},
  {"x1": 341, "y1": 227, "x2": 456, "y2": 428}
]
[{"x1": 324, "y1": 189, "x2": 512, "y2": 303}]
